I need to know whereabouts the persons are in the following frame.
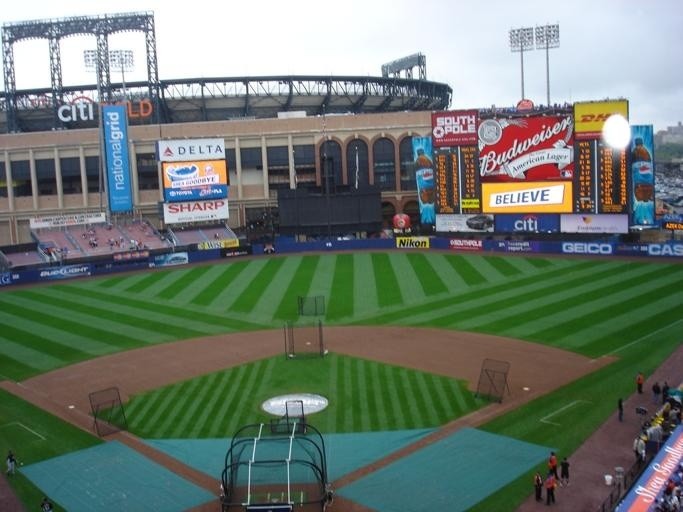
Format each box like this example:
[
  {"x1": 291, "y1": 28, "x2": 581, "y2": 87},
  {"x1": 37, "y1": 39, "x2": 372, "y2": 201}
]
[
  {"x1": 633, "y1": 370, "x2": 645, "y2": 395},
  {"x1": 39, "y1": 496, "x2": 53, "y2": 512},
  {"x1": 662, "y1": 380, "x2": 670, "y2": 403},
  {"x1": 558, "y1": 457, "x2": 571, "y2": 487},
  {"x1": 543, "y1": 472, "x2": 557, "y2": 505},
  {"x1": 633, "y1": 397, "x2": 682, "y2": 511},
  {"x1": 532, "y1": 470, "x2": 544, "y2": 502},
  {"x1": 4, "y1": 449, "x2": 18, "y2": 478},
  {"x1": 547, "y1": 450, "x2": 560, "y2": 480},
  {"x1": 79, "y1": 221, "x2": 145, "y2": 252},
  {"x1": 651, "y1": 380, "x2": 661, "y2": 403},
  {"x1": 617, "y1": 396, "x2": 625, "y2": 424}
]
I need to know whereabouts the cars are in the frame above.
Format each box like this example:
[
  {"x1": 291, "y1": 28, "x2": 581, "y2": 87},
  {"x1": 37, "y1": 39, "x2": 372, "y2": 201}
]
[{"x1": 655, "y1": 175, "x2": 683, "y2": 204}]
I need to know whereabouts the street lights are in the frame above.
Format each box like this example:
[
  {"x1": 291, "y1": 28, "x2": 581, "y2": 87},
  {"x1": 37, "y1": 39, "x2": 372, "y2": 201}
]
[
  {"x1": 533, "y1": 22, "x2": 560, "y2": 107},
  {"x1": 83, "y1": 47, "x2": 108, "y2": 101},
  {"x1": 508, "y1": 25, "x2": 534, "y2": 98},
  {"x1": 108, "y1": 49, "x2": 135, "y2": 100}
]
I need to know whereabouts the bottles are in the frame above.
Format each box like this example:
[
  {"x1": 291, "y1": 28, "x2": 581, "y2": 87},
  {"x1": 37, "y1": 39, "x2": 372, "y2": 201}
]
[
  {"x1": 414, "y1": 149, "x2": 434, "y2": 205},
  {"x1": 631, "y1": 137, "x2": 655, "y2": 202}
]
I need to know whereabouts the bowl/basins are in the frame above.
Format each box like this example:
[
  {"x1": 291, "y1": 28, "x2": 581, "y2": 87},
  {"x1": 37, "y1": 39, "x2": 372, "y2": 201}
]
[{"x1": 166, "y1": 164, "x2": 198, "y2": 182}]
